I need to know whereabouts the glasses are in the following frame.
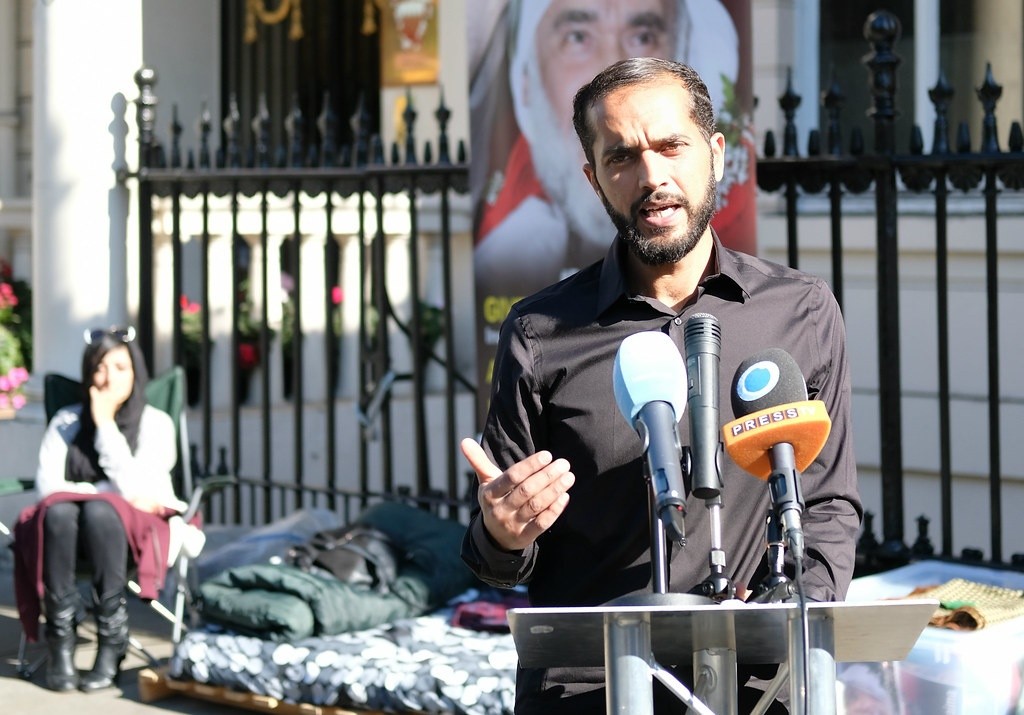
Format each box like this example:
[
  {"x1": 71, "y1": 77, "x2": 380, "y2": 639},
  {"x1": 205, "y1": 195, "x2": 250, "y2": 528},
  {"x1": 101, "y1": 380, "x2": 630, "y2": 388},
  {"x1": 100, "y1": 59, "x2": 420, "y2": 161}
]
[{"x1": 83, "y1": 325, "x2": 136, "y2": 345}]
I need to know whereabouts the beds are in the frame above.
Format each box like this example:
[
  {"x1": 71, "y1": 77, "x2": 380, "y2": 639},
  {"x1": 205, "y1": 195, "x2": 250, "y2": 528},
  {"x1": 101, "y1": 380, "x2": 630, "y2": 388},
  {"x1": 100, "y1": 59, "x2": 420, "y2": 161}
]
[{"x1": 138, "y1": 584, "x2": 518, "y2": 715}]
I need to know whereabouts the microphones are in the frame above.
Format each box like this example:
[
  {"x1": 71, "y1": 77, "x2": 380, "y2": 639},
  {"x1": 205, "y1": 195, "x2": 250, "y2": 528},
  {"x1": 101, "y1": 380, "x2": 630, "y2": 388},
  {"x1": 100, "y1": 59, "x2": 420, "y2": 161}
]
[
  {"x1": 723, "y1": 347, "x2": 831, "y2": 557},
  {"x1": 685, "y1": 313, "x2": 721, "y2": 499},
  {"x1": 613, "y1": 330, "x2": 688, "y2": 541}
]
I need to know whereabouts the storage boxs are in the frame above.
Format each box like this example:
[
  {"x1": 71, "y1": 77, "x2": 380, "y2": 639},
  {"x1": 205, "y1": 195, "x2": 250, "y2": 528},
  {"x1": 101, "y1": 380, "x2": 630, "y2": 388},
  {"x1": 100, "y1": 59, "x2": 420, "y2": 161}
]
[{"x1": 835, "y1": 562, "x2": 1024, "y2": 715}]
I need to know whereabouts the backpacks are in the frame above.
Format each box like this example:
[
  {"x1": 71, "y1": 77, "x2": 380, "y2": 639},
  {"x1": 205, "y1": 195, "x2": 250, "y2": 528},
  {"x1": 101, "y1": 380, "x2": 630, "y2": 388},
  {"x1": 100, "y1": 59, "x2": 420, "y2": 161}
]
[{"x1": 287, "y1": 522, "x2": 440, "y2": 598}]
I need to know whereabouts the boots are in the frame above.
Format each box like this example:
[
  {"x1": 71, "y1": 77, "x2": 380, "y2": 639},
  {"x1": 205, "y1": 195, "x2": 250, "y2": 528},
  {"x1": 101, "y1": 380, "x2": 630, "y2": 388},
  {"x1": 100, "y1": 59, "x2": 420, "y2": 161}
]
[
  {"x1": 44, "y1": 587, "x2": 82, "y2": 691},
  {"x1": 79, "y1": 585, "x2": 129, "y2": 692}
]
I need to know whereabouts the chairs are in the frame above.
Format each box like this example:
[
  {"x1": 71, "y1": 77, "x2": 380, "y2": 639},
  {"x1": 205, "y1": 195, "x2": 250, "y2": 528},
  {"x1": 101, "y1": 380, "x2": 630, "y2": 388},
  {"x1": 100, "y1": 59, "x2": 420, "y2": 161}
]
[{"x1": 0, "y1": 364, "x2": 237, "y2": 676}]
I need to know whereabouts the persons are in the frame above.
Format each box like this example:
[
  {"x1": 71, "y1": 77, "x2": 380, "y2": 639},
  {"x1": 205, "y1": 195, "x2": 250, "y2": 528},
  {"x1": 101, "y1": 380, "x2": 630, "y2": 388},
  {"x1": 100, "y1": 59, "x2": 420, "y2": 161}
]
[
  {"x1": 461, "y1": 54, "x2": 864, "y2": 714},
  {"x1": 35, "y1": 326, "x2": 189, "y2": 694},
  {"x1": 473, "y1": 0, "x2": 755, "y2": 291}
]
